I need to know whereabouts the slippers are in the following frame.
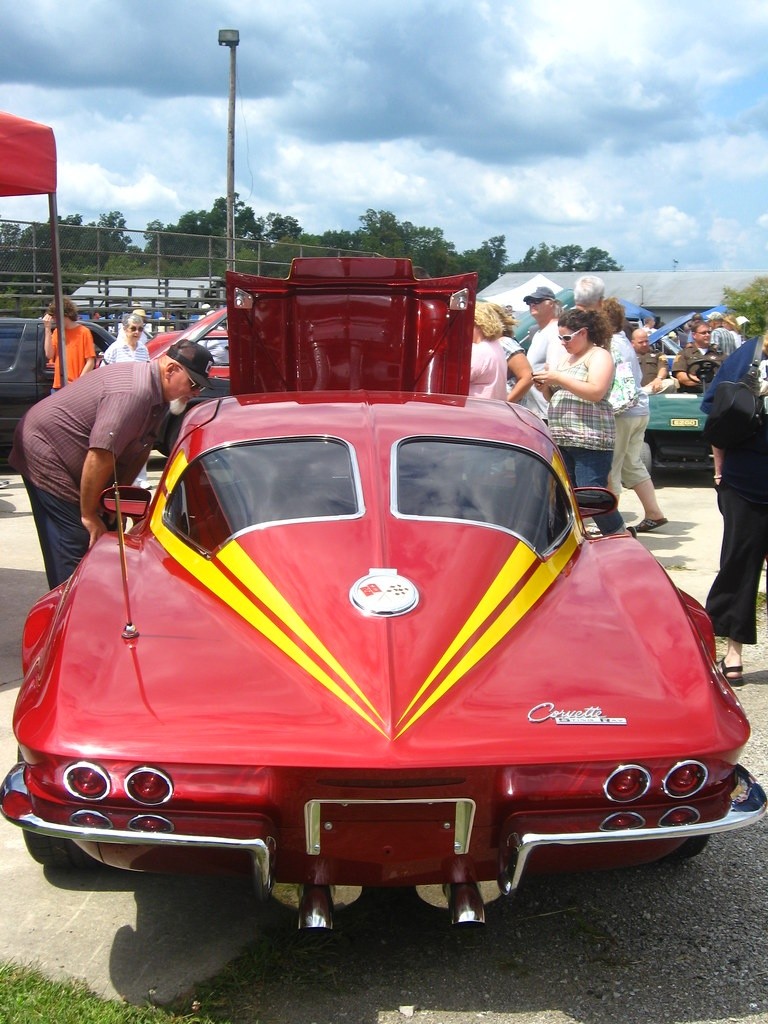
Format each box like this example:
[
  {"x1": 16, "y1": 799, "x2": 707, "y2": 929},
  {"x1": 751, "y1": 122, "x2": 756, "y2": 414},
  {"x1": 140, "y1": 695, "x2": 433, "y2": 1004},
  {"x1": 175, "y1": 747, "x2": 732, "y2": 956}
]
[{"x1": 633, "y1": 518, "x2": 667, "y2": 531}]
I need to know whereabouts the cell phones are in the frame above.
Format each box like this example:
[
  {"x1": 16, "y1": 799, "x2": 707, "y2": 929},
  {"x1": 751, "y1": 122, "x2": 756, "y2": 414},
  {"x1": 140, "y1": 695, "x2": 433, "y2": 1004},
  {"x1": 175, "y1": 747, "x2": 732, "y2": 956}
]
[{"x1": 531, "y1": 373, "x2": 544, "y2": 385}]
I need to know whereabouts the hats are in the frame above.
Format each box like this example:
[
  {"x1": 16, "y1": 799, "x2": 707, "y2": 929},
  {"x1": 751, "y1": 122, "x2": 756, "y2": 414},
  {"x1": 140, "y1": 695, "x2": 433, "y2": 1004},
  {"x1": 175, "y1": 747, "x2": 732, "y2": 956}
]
[
  {"x1": 133, "y1": 310, "x2": 146, "y2": 317},
  {"x1": 706, "y1": 312, "x2": 723, "y2": 320},
  {"x1": 523, "y1": 287, "x2": 555, "y2": 300},
  {"x1": 165, "y1": 339, "x2": 215, "y2": 390}
]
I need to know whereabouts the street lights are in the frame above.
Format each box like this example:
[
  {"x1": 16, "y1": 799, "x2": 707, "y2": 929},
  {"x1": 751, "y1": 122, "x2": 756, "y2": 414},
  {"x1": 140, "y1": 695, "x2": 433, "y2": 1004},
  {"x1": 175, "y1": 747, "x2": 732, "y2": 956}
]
[{"x1": 218, "y1": 29, "x2": 239, "y2": 273}]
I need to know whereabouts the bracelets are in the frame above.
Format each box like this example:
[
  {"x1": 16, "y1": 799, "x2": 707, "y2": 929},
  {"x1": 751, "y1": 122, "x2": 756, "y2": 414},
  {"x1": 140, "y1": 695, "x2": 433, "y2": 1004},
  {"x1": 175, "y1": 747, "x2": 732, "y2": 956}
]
[{"x1": 713, "y1": 475, "x2": 722, "y2": 479}]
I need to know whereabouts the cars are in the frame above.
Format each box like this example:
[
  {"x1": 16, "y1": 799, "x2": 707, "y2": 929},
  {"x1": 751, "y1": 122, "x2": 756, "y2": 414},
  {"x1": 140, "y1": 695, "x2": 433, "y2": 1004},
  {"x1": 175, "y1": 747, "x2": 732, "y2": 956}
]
[
  {"x1": 643, "y1": 356, "x2": 723, "y2": 472},
  {"x1": 141, "y1": 304, "x2": 231, "y2": 378}
]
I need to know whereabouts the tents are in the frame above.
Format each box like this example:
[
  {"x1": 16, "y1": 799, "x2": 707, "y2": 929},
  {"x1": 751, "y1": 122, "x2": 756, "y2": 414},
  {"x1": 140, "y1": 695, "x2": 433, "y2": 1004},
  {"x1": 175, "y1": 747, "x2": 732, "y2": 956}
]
[
  {"x1": 618, "y1": 297, "x2": 655, "y2": 328},
  {"x1": 0, "y1": 111, "x2": 67, "y2": 389}
]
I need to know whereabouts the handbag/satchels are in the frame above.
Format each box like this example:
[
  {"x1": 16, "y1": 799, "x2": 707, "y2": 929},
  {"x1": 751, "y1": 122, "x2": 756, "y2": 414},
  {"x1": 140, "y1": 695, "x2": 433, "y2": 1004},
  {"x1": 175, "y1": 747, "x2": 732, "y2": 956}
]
[{"x1": 704, "y1": 336, "x2": 764, "y2": 450}]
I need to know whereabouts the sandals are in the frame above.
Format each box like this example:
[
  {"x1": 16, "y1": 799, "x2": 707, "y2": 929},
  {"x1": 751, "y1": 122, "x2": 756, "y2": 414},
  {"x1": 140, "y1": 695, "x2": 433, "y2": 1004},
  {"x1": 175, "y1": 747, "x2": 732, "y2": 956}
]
[{"x1": 715, "y1": 657, "x2": 743, "y2": 686}]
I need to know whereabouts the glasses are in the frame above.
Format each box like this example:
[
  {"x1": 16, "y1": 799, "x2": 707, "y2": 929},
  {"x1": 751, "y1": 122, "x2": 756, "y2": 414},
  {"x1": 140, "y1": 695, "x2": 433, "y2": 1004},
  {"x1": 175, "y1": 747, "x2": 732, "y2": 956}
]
[
  {"x1": 526, "y1": 298, "x2": 547, "y2": 305},
  {"x1": 696, "y1": 331, "x2": 711, "y2": 335},
  {"x1": 129, "y1": 326, "x2": 143, "y2": 332},
  {"x1": 184, "y1": 371, "x2": 206, "y2": 392},
  {"x1": 558, "y1": 327, "x2": 588, "y2": 342}
]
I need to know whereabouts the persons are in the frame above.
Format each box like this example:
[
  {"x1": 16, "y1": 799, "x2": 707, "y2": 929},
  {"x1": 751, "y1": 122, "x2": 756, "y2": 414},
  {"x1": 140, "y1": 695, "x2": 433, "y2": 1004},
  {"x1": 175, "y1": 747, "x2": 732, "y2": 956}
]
[
  {"x1": 41, "y1": 295, "x2": 97, "y2": 396},
  {"x1": 699, "y1": 324, "x2": 768, "y2": 688},
  {"x1": 469, "y1": 300, "x2": 509, "y2": 402},
  {"x1": 92, "y1": 312, "x2": 100, "y2": 319},
  {"x1": 497, "y1": 307, "x2": 549, "y2": 425},
  {"x1": 522, "y1": 275, "x2": 670, "y2": 536},
  {"x1": 621, "y1": 311, "x2": 741, "y2": 396},
  {"x1": 116, "y1": 309, "x2": 149, "y2": 344},
  {"x1": 6, "y1": 339, "x2": 217, "y2": 591},
  {"x1": 99, "y1": 313, "x2": 153, "y2": 490},
  {"x1": 153, "y1": 303, "x2": 216, "y2": 331}
]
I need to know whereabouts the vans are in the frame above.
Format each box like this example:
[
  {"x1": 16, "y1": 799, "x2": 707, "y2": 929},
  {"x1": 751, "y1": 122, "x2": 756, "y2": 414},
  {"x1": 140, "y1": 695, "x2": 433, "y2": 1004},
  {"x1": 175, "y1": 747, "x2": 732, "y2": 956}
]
[{"x1": 0, "y1": 317, "x2": 223, "y2": 459}]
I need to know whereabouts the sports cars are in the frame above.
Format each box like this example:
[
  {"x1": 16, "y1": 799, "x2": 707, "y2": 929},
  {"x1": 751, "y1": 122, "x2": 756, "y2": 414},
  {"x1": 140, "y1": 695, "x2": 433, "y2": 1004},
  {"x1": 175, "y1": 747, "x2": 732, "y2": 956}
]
[{"x1": 0, "y1": 256, "x2": 767, "y2": 932}]
